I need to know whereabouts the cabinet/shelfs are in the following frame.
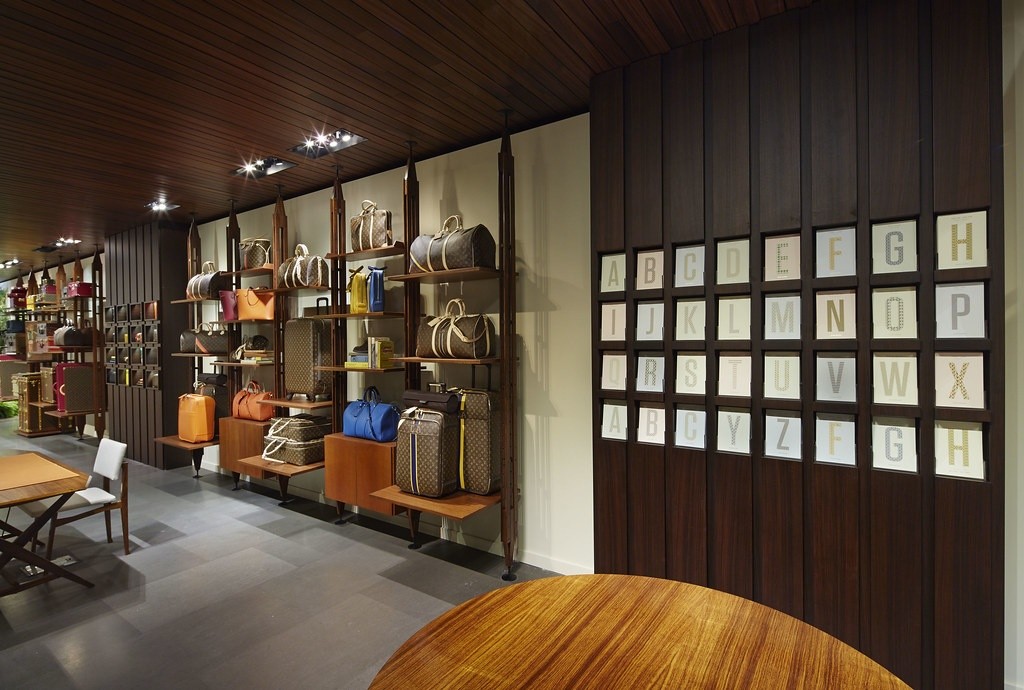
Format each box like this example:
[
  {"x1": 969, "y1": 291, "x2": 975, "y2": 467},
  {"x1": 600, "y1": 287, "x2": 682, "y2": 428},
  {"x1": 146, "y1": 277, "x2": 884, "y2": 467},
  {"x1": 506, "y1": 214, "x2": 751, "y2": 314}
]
[
  {"x1": 153, "y1": 107, "x2": 521, "y2": 582},
  {"x1": 0, "y1": 243, "x2": 106, "y2": 448},
  {"x1": 103, "y1": 220, "x2": 194, "y2": 472}
]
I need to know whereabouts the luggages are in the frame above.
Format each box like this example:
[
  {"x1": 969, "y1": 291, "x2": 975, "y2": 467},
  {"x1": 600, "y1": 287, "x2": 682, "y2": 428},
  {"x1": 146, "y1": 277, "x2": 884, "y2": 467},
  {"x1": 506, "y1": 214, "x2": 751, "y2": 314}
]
[
  {"x1": 444, "y1": 364, "x2": 502, "y2": 496},
  {"x1": 195, "y1": 361, "x2": 230, "y2": 437},
  {"x1": 178, "y1": 393, "x2": 215, "y2": 443},
  {"x1": 395, "y1": 382, "x2": 459, "y2": 497},
  {"x1": 285, "y1": 297, "x2": 333, "y2": 403}
]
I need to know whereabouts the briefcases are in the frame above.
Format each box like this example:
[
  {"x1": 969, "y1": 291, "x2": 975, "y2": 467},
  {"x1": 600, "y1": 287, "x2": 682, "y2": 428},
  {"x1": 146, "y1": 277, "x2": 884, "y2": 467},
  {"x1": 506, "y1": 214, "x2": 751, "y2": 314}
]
[
  {"x1": 67, "y1": 281, "x2": 92, "y2": 297},
  {"x1": 16, "y1": 359, "x2": 103, "y2": 433}
]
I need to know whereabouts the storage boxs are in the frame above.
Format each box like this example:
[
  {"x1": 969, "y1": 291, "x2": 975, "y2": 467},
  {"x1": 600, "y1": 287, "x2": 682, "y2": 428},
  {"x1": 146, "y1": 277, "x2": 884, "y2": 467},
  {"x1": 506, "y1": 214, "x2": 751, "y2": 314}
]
[
  {"x1": 42, "y1": 284, "x2": 56, "y2": 294},
  {"x1": 40, "y1": 367, "x2": 57, "y2": 404},
  {"x1": 16, "y1": 372, "x2": 75, "y2": 434},
  {"x1": 67, "y1": 281, "x2": 92, "y2": 296},
  {"x1": 41, "y1": 294, "x2": 57, "y2": 302},
  {"x1": 26, "y1": 295, "x2": 51, "y2": 311},
  {"x1": 7, "y1": 293, "x2": 26, "y2": 308},
  {"x1": 23, "y1": 320, "x2": 63, "y2": 360},
  {"x1": 5, "y1": 333, "x2": 26, "y2": 355}
]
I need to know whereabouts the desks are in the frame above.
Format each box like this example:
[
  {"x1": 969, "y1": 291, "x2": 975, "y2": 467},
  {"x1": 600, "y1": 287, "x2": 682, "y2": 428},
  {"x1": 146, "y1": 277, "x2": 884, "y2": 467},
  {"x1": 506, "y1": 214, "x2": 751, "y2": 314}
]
[
  {"x1": 369, "y1": 574, "x2": 914, "y2": 690},
  {"x1": 0, "y1": 451, "x2": 96, "y2": 588}
]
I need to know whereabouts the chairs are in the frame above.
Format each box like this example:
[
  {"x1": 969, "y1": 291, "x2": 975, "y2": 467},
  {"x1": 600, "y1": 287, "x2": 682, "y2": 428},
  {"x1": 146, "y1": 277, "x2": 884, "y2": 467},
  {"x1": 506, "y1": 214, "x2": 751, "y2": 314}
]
[{"x1": 20, "y1": 437, "x2": 130, "y2": 576}]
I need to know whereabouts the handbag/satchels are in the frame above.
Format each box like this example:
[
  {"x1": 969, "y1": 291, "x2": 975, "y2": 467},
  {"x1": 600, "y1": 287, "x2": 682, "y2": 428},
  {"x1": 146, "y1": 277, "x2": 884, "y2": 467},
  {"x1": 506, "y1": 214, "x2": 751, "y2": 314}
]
[
  {"x1": 53, "y1": 318, "x2": 83, "y2": 345},
  {"x1": 365, "y1": 265, "x2": 388, "y2": 312},
  {"x1": 267, "y1": 413, "x2": 332, "y2": 442},
  {"x1": 195, "y1": 322, "x2": 228, "y2": 354},
  {"x1": 343, "y1": 385, "x2": 401, "y2": 443},
  {"x1": 277, "y1": 244, "x2": 329, "y2": 286},
  {"x1": 236, "y1": 286, "x2": 274, "y2": 320},
  {"x1": 233, "y1": 381, "x2": 274, "y2": 421},
  {"x1": 261, "y1": 435, "x2": 325, "y2": 466},
  {"x1": 186, "y1": 261, "x2": 228, "y2": 298},
  {"x1": 350, "y1": 199, "x2": 393, "y2": 252},
  {"x1": 239, "y1": 238, "x2": 271, "y2": 269},
  {"x1": 80, "y1": 319, "x2": 100, "y2": 345},
  {"x1": 416, "y1": 298, "x2": 495, "y2": 359},
  {"x1": 346, "y1": 266, "x2": 368, "y2": 314},
  {"x1": 197, "y1": 373, "x2": 227, "y2": 385},
  {"x1": 409, "y1": 214, "x2": 497, "y2": 273},
  {"x1": 219, "y1": 291, "x2": 238, "y2": 321},
  {"x1": 232, "y1": 335, "x2": 269, "y2": 360},
  {"x1": 180, "y1": 329, "x2": 195, "y2": 353}
]
